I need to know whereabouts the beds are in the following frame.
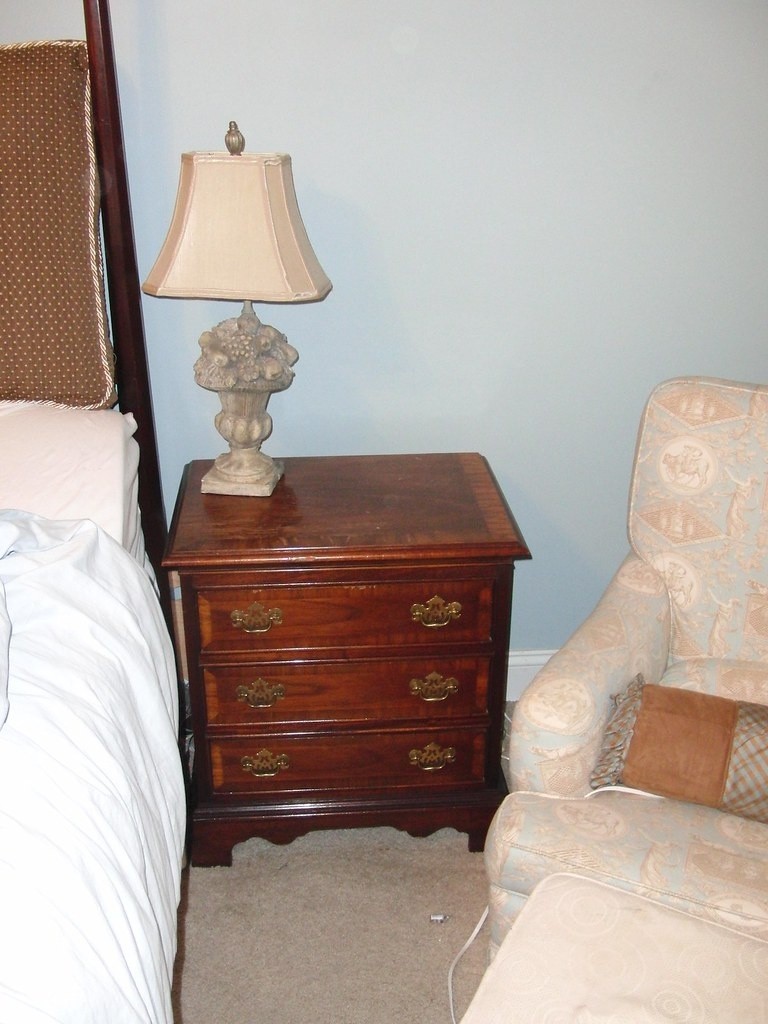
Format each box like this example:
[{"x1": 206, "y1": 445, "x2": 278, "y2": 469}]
[{"x1": 0, "y1": 0, "x2": 190, "y2": 1024}]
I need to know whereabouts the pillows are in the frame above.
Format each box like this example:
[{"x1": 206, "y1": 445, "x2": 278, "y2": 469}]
[{"x1": 0, "y1": 408, "x2": 147, "y2": 550}]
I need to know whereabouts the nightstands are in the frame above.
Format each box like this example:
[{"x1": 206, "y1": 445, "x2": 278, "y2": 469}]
[{"x1": 166, "y1": 447, "x2": 534, "y2": 866}]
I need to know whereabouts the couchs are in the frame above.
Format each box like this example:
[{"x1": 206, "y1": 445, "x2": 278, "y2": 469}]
[{"x1": 483, "y1": 376, "x2": 768, "y2": 969}]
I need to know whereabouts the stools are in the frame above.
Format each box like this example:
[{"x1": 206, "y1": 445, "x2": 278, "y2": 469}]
[{"x1": 433, "y1": 869, "x2": 768, "y2": 1024}]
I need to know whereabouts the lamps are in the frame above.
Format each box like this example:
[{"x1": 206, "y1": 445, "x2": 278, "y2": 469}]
[{"x1": 141, "y1": 118, "x2": 333, "y2": 499}]
